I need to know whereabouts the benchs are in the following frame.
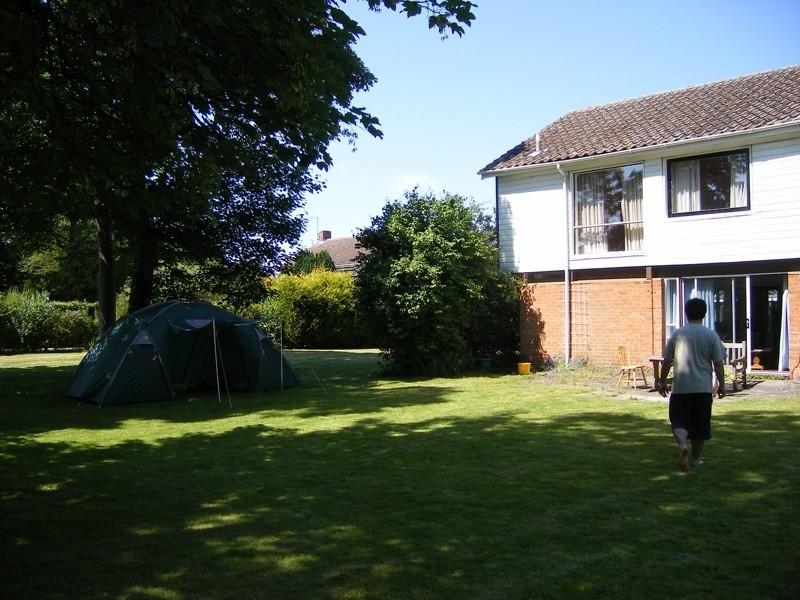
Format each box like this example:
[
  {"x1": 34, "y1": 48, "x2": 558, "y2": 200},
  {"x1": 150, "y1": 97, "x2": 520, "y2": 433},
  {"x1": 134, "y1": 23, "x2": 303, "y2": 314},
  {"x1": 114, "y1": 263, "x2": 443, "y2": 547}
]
[{"x1": 649, "y1": 341, "x2": 747, "y2": 390}]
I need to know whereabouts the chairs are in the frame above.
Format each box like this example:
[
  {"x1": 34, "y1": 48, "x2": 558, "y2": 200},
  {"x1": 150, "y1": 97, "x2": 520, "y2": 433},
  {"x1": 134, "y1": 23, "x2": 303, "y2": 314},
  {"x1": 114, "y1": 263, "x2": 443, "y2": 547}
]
[{"x1": 615, "y1": 346, "x2": 648, "y2": 391}]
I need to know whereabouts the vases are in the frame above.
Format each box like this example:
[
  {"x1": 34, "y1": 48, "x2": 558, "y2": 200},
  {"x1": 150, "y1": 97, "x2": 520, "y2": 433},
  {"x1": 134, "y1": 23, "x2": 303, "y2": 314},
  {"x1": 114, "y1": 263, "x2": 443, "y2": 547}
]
[{"x1": 517, "y1": 362, "x2": 532, "y2": 374}]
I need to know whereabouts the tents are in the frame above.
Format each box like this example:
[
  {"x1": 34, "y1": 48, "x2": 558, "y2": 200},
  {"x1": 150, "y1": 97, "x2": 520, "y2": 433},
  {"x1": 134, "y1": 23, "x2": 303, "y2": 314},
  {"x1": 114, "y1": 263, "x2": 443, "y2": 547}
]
[{"x1": 65, "y1": 299, "x2": 303, "y2": 406}]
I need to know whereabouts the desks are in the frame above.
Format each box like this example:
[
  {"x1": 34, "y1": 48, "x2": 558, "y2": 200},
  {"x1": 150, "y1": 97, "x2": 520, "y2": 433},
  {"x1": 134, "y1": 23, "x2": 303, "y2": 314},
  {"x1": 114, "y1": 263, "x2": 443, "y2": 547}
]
[{"x1": 752, "y1": 349, "x2": 777, "y2": 369}]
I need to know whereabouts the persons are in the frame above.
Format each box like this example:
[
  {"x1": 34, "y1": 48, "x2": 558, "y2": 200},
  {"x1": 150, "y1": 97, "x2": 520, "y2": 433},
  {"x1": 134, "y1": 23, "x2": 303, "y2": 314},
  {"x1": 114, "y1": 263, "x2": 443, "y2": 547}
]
[{"x1": 658, "y1": 299, "x2": 726, "y2": 469}]
